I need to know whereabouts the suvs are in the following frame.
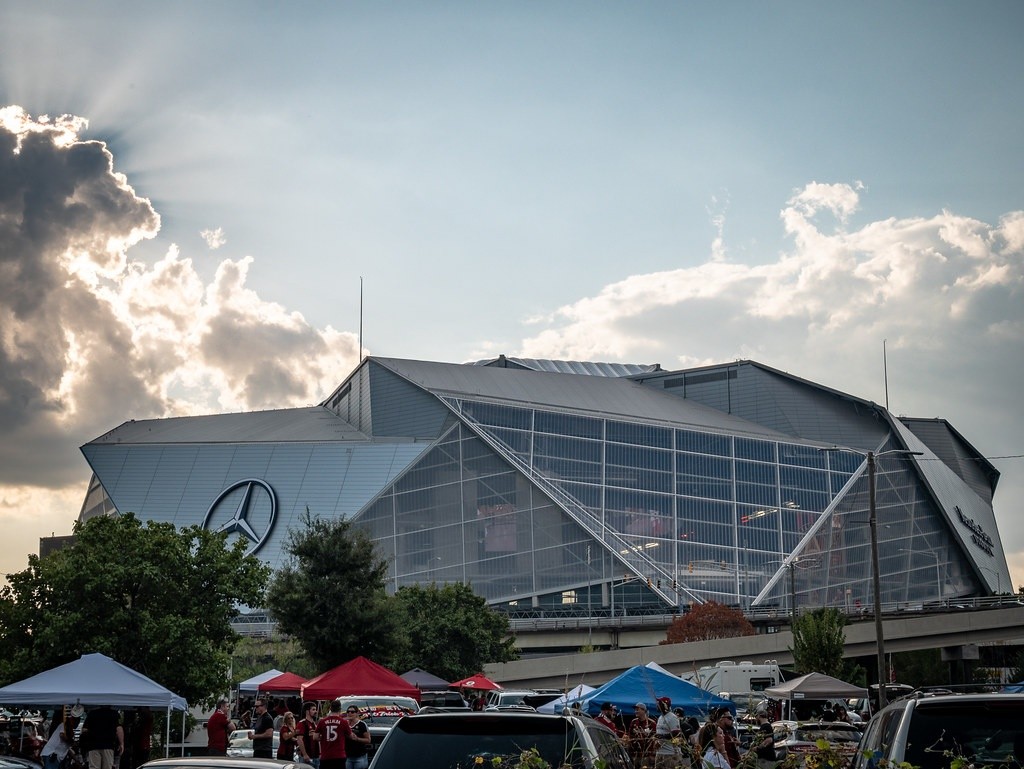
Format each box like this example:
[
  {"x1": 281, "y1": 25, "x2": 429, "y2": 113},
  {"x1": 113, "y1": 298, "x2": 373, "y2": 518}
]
[
  {"x1": 368, "y1": 705, "x2": 636, "y2": 769},
  {"x1": 417, "y1": 688, "x2": 566, "y2": 715},
  {"x1": 850, "y1": 683, "x2": 1024, "y2": 769},
  {"x1": 326, "y1": 694, "x2": 420, "y2": 758}
]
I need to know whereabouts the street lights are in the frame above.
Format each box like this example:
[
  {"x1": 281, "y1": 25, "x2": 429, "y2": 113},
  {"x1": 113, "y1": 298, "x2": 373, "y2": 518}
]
[
  {"x1": 977, "y1": 566, "x2": 1002, "y2": 606},
  {"x1": 762, "y1": 558, "x2": 823, "y2": 628},
  {"x1": 427, "y1": 555, "x2": 441, "y2": 584},
  {"x1": 898, "y1": 549, "x2": 942, "y2": 607},
  {"x1": 865, "y1": 524, "x2": 892, "y2": 607},
  {"x1": 817, "y1": 447, "x2": 925, "y2": 708}
]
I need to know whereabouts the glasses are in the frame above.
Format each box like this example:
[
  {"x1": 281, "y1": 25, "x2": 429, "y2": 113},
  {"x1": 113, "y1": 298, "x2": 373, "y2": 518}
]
[
  {"x1": 288, "y1": 718, "x2": 293, "y2": 719},
  {"x1": 722, "y1": 716, "x2": 732, "y2": 720},
  {"x1": 256, "y1": 705, "x2": 264, "y2": 708},
  {"x1": 347, "y1": 710, "x2": 356, "y2": 714}
]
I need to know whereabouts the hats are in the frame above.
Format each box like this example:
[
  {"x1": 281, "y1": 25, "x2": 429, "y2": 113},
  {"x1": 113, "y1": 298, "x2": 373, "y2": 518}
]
[
  {"x1": 657, "y1": 697, "x2": 671, "y2": 707},
  {"x1": 601, "y1": 702, "x2": 612, "y2": 710},
  {"x1": 632, "y1": 702, "x2": 648, "y2": 713},
  {"x1": 759, "y1": 710, "x2": 768, "y2": 717},
  {"x1": 673, "y1": 707, "x2": 685, "y2": 717}
]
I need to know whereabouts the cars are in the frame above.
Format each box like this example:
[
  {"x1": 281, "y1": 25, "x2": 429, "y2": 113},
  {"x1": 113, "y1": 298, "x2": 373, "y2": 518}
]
[
  {"x1": 136, "y1": 756, "x2": 315, "y2": 769},
  {"x1": 922, "y1": 600, "x2": 974, "y2": 610},
  {"x1": 488, "y1": 600, "x2": 778, "y2": 619},
  {"x1": 226, "y1": 729, "x2": 300, "y2": 763},
  {"x1": 770, "y1": 720, "x2": 863, "y2": 769},
  {"x1": 714, "y1": 682, "x2": 1024, "y2": 736},
  {"x1": 990, "y1": 601, "x2": 1024, "y2": 607}
]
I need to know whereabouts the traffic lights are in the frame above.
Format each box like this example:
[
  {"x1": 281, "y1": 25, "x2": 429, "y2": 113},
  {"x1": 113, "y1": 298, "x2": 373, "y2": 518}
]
[
  {"x1": 689, "y1": 563, "x2": 694, "y2": 573},
  {"x1": 672, "y1": 580, "x2": 676, "y2": 589},
  {"x1": 646, "y1": 579, "x2": 651, "y2": 587},
  {"x1": 624, "y1": 574, "x2": 629, "y2": 582},
  {"x1": 657, "y1": 580, "x2": 661, "y2": 589}
]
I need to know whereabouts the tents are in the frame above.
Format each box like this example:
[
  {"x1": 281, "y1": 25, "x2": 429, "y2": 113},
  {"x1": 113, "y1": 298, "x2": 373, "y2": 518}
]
[
  {"x1": 536, "y1": 683, "x2": 597, "y2": 714},
  {"x1": 449, "y1": 674, "x2": 506, "y2": 693},
  {"x1": 552, "y1": 665, "x2": 737, "y2": 723},
  {"x1": 0, "y1": 652, "x2": 187, "y2": 769},
  {"x1": 253, "y1": 672, "x2": 308, "y2": 719},
  {"x1": 646, "y1": 662, "x2": 695, "y2": 686},
  {"x1": 301, "y1": 657, "x2": 421, "y2": 707},
  {"x1": 397, "y1": 668, "x2": 448, "y2": 690},
  {"x1": 764, "y1": 672, "x2": 873, "y2": 721},
  {"x1": 235, "y1": 669, "x2": 285, "y2": 716}
]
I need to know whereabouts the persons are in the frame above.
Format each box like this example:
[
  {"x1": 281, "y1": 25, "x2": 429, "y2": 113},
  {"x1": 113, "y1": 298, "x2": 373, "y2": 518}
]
[
  {"x1": 627, "y1": 704, "x2": 657, "y2": 765},
  {"x1": 593, "y1": 702, "x2": 617, "y2": 736},
  {"x1": 206, "y1": 700, "x2": 236, "y2": 756},
  {"x1": 655, "y1": 697, "x2": 740, "y2": 769},
  {"x1": 821, "y1": 702, "x2": 852, "y2": 725},
  {"x1": 749, "y1": 722, "x2": 777, "y2": 761},
  {"x1": 40, "y1": 702, "x2": 152, "y2": 769},
  {"x1": 233, "y1": 697, "x2": 373, "y2": 769},
  {"x1": 571, "y1": 703, "x2": 582, "y2": 716}
]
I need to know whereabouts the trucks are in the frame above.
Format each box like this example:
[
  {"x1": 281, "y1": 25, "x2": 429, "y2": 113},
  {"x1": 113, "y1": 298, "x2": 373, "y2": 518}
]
[{"x1": 681, "y1": 659, "x2": 780, "y2": 695}]
